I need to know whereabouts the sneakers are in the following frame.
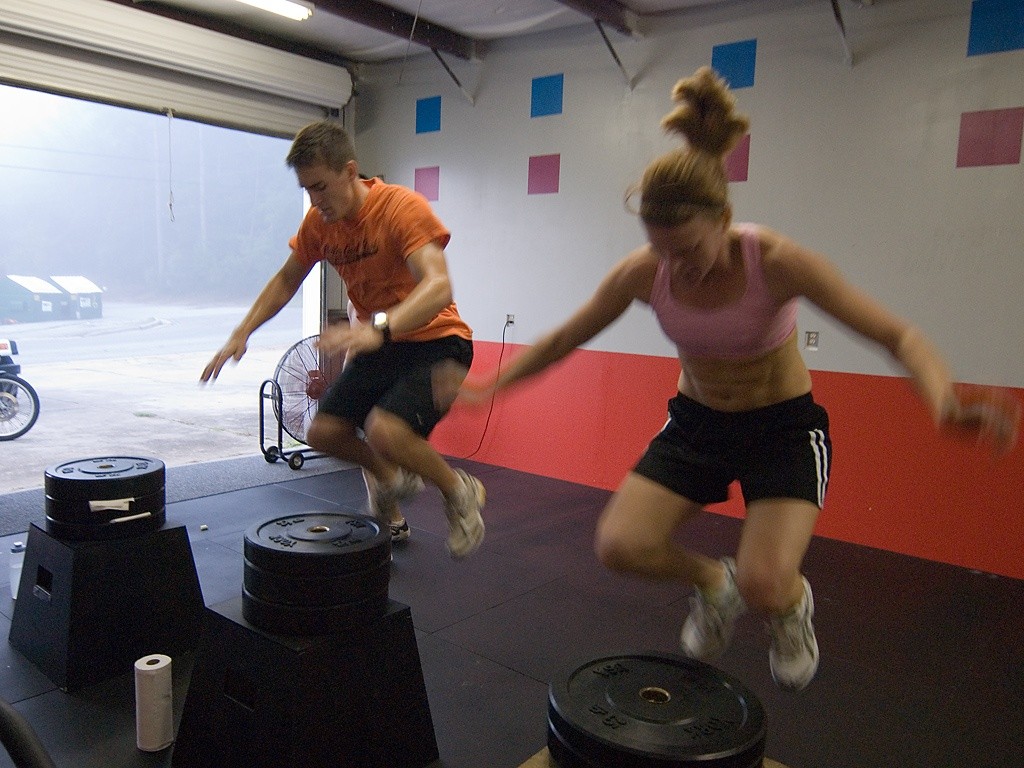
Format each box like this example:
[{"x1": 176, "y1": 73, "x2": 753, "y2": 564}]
[
  {"x1": 387, "y1": 517, "x2": 410, "y2": 541},
  {"x1": 765, "y1": 573, "x2": 819, "y2": 690},
  {"x1": 382, "y1": 465, "x2": 426, "y2": 501},
  {"x1": 440, "y1": 467, "x2": 486, "y2": 558},
  {"x1": 679, "y1": 556, "x2": 747, "y2": 662}
]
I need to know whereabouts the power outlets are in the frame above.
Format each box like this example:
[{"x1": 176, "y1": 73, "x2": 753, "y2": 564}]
[
  {"x1": 506, "y1": 314, "x2": 513, "y2": 328},
  {"x1": 807, "y1": 332, "x2": 818, "y2": 347}
]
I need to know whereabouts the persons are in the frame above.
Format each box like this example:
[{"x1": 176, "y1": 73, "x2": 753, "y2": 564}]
[
  {"x1": 334, "y1": 287, "x2": 413, "y2": 546},
  {"x1": 201, "y1": 123, "x2": 487, "y2": 562},
  {"x1": 429, "y1": 67, "x2": 1022, "y2": 689}
]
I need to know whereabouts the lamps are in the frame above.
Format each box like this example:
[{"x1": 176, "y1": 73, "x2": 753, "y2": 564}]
[{"x1": 233, "y1": 0, "x2": 318, "y2": 23}]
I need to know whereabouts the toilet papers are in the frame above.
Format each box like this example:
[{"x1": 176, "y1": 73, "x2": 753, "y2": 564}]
[{"x1": 132, "y1": 654, "x2": 176, "y2": 754}]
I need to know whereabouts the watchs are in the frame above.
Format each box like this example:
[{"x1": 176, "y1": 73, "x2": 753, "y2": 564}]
[{"x1": 370, "y1": 309, "x2": 392, "y2": 345}]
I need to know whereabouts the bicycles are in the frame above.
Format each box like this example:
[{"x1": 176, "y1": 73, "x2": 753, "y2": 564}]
[{"x1": 0, "y1": 339, "x2": 41, "y2": 441}]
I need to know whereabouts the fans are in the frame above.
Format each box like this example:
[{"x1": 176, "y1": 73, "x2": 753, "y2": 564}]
[{"x1": 271, "y1": 335, "x2": 343, "y2": 450}]
